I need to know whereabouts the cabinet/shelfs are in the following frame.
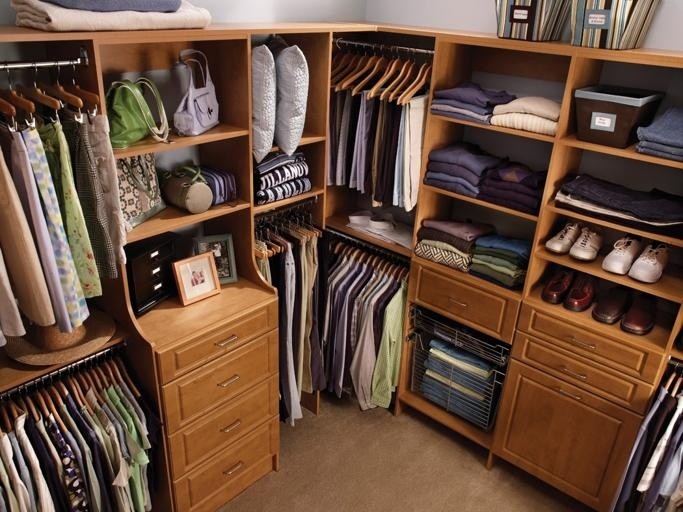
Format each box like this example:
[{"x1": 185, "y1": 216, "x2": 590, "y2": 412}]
[
  {"x1": 121, "y1": 230, "x2": 177, "y2": 320},
  {"x1": 0, "y1": 22, "x2": 683, "y2": 512}
]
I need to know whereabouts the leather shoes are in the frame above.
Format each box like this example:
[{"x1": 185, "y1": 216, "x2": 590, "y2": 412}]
[
  {"x1": 620, "y1": 294, "x2": 658, "y2": 335},
  {"x1": 563, "y1": 270, "x2": 598, "y2": 311},
  {"x1": 591, "y1": 285, "x2": 626, "y2": 324},
  {"x1": 540, "y1": 266, "x2": 574, "y2": 304}
]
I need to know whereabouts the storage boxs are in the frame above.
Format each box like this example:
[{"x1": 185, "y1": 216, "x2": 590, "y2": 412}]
[
  {"x1": 492, "y1": 0, "x2": 570, "y2": 44},
  {"x1": 571, "y1": 85, "x2": 667, "y2": 150},
  {"x1": 566, "y1": 0, "x2": 661, "y2": 50}
]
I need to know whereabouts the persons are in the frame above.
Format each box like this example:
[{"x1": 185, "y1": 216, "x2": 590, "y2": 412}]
[
  {"x1": 190, "y1": 271, "x2": 199, "y2": 286},
  {"x1": 198, "y1": 271, "x2": 206, "y2": 284},
  {"x1": 212, "y1": 243, "x2": 223, "y2": 269}
]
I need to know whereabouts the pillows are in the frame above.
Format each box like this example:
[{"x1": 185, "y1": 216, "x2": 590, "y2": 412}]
[
  {"x1": 274, "y1": 43, "x2": 309, "y2": 159},
  {"x1": 249, "y1": 44, "x2": 275, "y2": 165}
]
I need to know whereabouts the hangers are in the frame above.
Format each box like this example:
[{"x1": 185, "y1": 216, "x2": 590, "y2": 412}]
[
  {"x1": 329, "y1": 40, "x2": 433, "y2": 108},
  {"x1": 0, "y1": 57, "x2": 102, "y2": 133},
  {"x1": 326, "y1": 232, "x2": 408, "y2": 287},
  {"x1": 654, "y1": 363, "x2": 682, "y2": 409},
  {"x1": 0, "y1": 344, "x2": 142, "y2": 435},
  {"x1": 254, "y1": 200, "x2": 322, "y2": 260}
]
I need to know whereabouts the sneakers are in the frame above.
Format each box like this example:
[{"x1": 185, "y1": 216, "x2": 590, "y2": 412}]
[
  {"x1": 601, "y1": 235, "x2": 641, "y2": 275},
  {"x1": 545, "y1": 222, "x2": 582, "y2": 254},
  {"x1": 629, "y1": 242, "x2": 670, "y2": 282},
  {"x1": 570, "y1": 226, "x2": 603, "y2": 263}
]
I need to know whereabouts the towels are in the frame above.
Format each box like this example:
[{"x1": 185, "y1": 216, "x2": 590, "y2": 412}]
[
  {"x1": 10, "y1": 2, "x2": 212, "y2": 30},
  {"x1": 45, "y1": 0, "x2": 182, "y2": 13}
]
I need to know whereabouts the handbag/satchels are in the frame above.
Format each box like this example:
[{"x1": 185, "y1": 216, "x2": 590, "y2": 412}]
[
  {"x1": 104, "y1": 77, "x2": 170, "y2": 149},
  {"x1": 174, "y1": 49, "x2": 221, "y2": 136},
  {"x1": 160, "y1": 162, "x2": 217, "y2": 214}
]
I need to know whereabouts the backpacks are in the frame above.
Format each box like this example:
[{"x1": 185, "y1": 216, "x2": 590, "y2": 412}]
[{"x1": 116, "y1": 152, "x2": 168, "y2": 231}]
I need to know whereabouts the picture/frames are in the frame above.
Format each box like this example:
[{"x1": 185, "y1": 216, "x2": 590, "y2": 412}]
[
  {"x1": 170, "y1": 251, "x2": 221, "y2": 308},
  {"x1": 190, "y1": 233, "x2": 239, "y2": 286}
]
